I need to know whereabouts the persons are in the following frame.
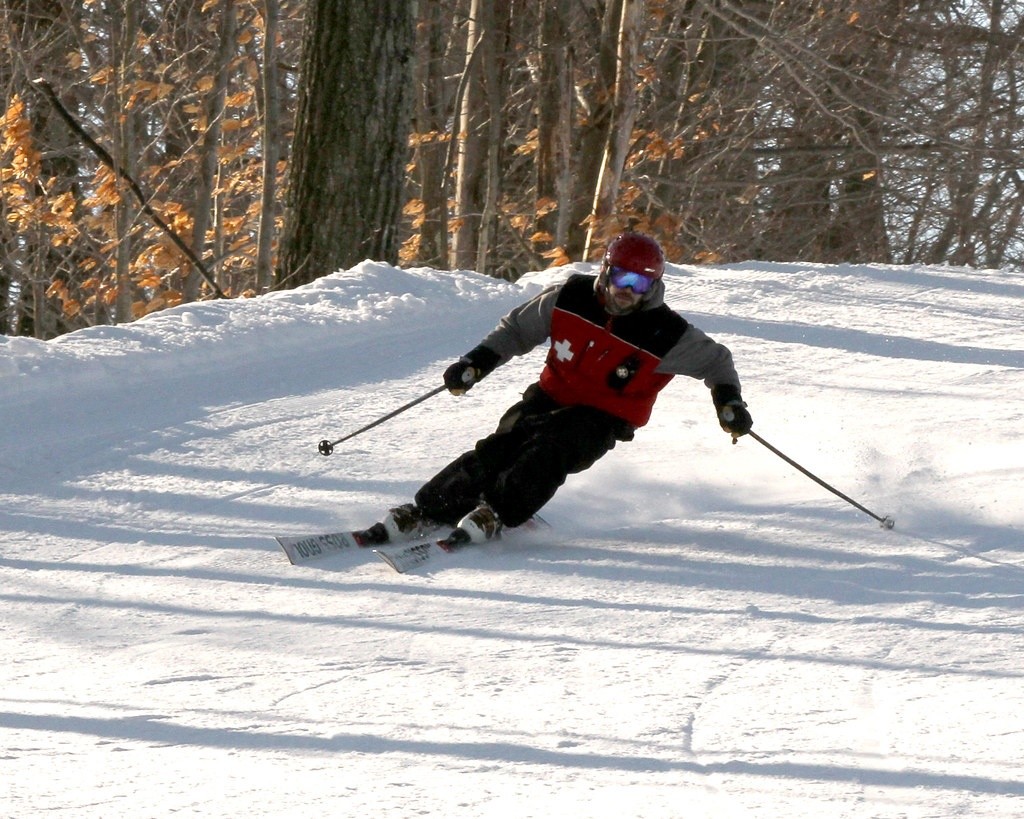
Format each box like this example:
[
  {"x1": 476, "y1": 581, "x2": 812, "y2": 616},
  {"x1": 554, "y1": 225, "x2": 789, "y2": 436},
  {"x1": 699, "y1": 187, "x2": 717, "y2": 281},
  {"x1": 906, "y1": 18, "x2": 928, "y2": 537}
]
[{"x1": 381, "y1": 232, "x2": 753, "y2": 544}]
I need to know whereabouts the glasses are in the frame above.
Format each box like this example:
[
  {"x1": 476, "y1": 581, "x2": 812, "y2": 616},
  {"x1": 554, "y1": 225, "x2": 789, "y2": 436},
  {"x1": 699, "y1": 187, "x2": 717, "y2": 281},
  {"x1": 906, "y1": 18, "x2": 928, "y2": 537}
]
[{"x1": 606, "y1": 266, "x2": 654, "y2": 294}]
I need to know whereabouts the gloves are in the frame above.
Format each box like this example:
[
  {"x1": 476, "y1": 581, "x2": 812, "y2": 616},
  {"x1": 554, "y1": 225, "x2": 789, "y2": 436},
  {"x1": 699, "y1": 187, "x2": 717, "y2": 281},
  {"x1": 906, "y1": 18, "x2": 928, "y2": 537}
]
[
  {"x1": 443, "y1": 356, "x2": 481, "y2": 395},
  {"x1": 712, "y1": 384, "x2": 752, "y2": 439}
]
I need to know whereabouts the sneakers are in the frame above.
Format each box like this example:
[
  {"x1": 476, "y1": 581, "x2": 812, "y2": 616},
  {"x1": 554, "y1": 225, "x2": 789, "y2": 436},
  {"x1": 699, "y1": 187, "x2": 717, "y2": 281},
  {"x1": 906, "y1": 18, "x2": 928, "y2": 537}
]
[
  {"x1": 456, "y1": 502, "x2": 502, "y2": 545},
  {"x1": 384, "y1": 502, "x2": 436, "y2": 541}
]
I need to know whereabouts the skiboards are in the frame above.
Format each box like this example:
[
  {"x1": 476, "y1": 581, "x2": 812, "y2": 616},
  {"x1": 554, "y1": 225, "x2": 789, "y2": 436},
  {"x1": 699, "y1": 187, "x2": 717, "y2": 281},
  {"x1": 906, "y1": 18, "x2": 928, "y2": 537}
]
[{"x1": 274, "y1": 511, "x2": 554, "y2": 574}]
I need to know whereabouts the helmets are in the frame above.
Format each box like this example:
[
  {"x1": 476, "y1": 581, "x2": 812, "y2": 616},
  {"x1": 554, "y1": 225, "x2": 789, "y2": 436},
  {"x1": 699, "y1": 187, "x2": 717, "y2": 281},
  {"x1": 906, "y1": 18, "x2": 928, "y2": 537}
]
[{"x1": 604, "y1": 231, "x2": 666, "y2": 283}]
